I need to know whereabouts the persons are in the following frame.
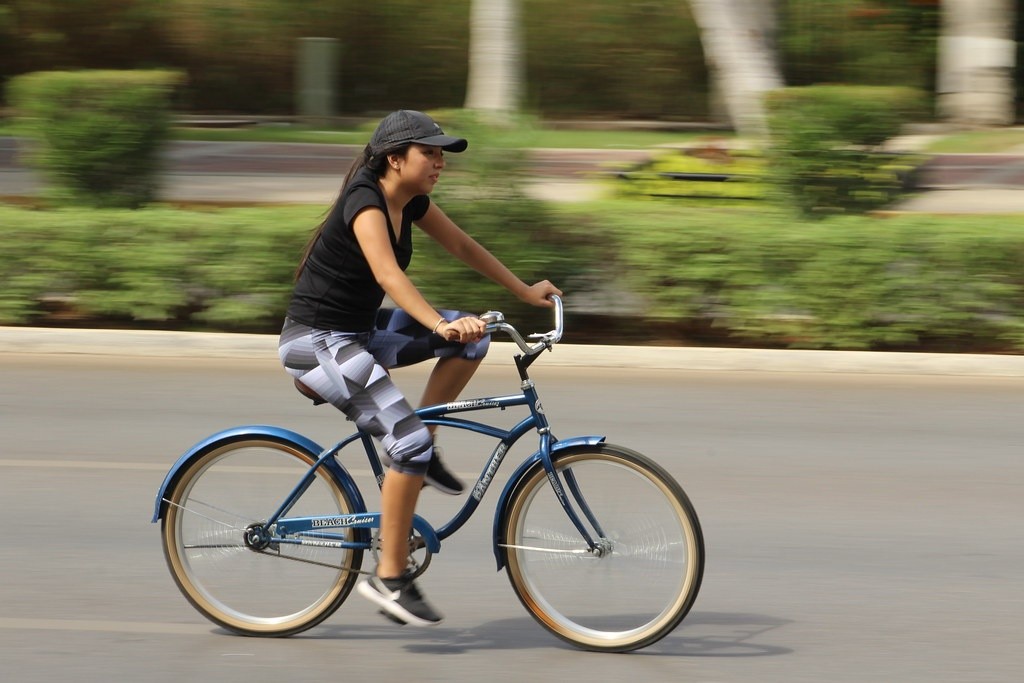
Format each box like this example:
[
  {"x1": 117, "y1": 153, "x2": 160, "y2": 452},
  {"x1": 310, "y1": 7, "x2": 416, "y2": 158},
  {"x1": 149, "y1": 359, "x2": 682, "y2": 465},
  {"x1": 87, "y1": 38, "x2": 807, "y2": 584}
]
[{"x1": 276, "y1": 109, "x2": 563, "y2": 625}]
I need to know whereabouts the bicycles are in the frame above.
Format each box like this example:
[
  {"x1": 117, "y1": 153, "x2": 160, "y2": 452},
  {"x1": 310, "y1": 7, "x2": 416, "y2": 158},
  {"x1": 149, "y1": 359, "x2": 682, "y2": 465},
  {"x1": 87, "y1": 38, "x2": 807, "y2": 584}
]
[{"x1": 150, "y1": 294, "x2": 708, "y2": 655}]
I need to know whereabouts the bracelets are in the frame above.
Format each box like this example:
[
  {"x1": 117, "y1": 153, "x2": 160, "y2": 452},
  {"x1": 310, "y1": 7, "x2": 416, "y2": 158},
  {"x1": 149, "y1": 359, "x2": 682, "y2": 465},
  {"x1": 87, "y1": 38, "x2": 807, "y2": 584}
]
[{"x1": 433, "y1": 318, "x2": 446, "y2": 335}]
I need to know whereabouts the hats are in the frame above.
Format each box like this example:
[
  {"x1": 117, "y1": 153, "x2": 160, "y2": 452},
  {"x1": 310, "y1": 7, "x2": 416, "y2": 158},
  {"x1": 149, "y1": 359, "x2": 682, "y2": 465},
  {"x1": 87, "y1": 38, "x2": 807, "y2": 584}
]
[{"x1": 370, "y1": 110, "x2": 468, "y2": 153}]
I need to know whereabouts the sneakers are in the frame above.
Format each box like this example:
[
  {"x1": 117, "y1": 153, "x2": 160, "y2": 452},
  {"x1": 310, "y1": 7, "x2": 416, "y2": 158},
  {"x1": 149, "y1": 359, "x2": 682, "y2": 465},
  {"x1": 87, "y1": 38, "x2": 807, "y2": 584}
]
[
  {"x1": 357, "y1": 569, "x2": 444, "y2": 627},
  {"x1": 381, "y1": 434, "x2": 463, "y2": 495}
]
